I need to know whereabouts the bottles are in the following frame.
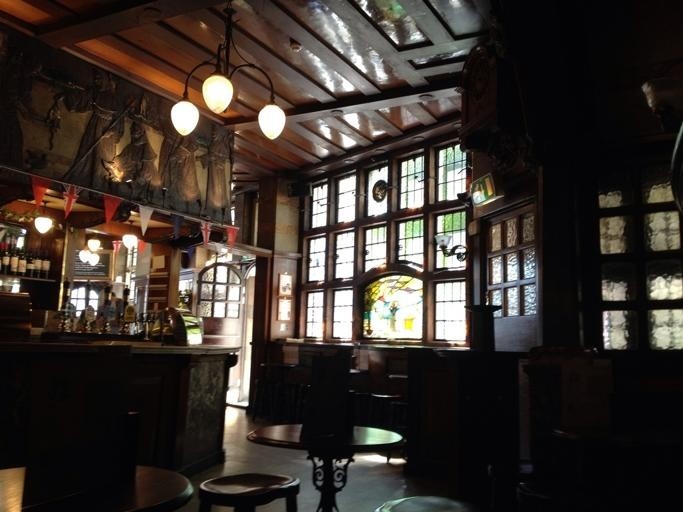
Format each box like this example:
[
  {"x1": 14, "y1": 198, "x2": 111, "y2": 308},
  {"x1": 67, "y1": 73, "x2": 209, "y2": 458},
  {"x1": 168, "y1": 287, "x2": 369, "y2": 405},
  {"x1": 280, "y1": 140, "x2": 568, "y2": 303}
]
[{"x1": 0, "y1": 248, "x2": 51, "y2": 280}]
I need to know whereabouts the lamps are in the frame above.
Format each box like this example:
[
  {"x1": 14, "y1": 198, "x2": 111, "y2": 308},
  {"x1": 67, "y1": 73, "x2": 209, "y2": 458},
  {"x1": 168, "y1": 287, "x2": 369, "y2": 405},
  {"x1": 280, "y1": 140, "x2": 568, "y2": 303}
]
[
  {"x1": 638, "y1": 80, "x2": 659, "y2": 113},
  {"x1": 34, "y1": 200, "x2": 55, "y2": 234},
  {"x1": 373, "y1": 180, "x2": 393, "y2": 202},
  {"x1": 79, "y1": 235, "x2": 101, "y2": 267},
  {"x1": 122, "y1": 233, "x2": 139, "y2": 250},
  {"x1": 170, "y1": 0, "x2": 286, "y2": 141}
]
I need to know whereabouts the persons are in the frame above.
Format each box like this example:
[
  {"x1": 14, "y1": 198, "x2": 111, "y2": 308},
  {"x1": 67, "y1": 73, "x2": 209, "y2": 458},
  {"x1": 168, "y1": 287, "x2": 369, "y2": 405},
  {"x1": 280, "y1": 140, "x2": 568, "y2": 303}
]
[{"x1": 0, "y1": 58, "x2": 231, "y2": 227}]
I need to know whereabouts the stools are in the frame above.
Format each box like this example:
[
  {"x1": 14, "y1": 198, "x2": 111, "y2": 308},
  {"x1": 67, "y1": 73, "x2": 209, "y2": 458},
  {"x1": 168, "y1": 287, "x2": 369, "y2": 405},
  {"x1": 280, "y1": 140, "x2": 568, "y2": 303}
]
[
  {"x1": 198, "y1": 472, "x2": 300, "y2": 512},
  {"x1": 374, "y1": 495, "x2": 472, "y2": 512}
]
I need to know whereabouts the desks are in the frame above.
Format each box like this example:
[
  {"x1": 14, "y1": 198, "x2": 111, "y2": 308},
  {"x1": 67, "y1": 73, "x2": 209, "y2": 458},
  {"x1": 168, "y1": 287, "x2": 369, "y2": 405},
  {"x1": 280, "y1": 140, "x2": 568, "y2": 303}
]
[
  {"x1": 1, "y1": 466, "x2": 193, "y2": 511},
  {"x1": 247, "y1": 424, "x2": 404, "y2": 512}
]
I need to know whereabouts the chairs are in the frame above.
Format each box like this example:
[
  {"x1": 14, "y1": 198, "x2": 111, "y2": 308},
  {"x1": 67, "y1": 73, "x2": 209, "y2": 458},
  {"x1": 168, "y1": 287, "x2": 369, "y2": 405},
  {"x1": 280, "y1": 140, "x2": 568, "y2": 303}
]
[
  {"x1": 486, "y1": 427, "x2": 615, "y2": 512},
  {"x1": 251, "y1": 362, "x2": 417, "y2": 466}
]
